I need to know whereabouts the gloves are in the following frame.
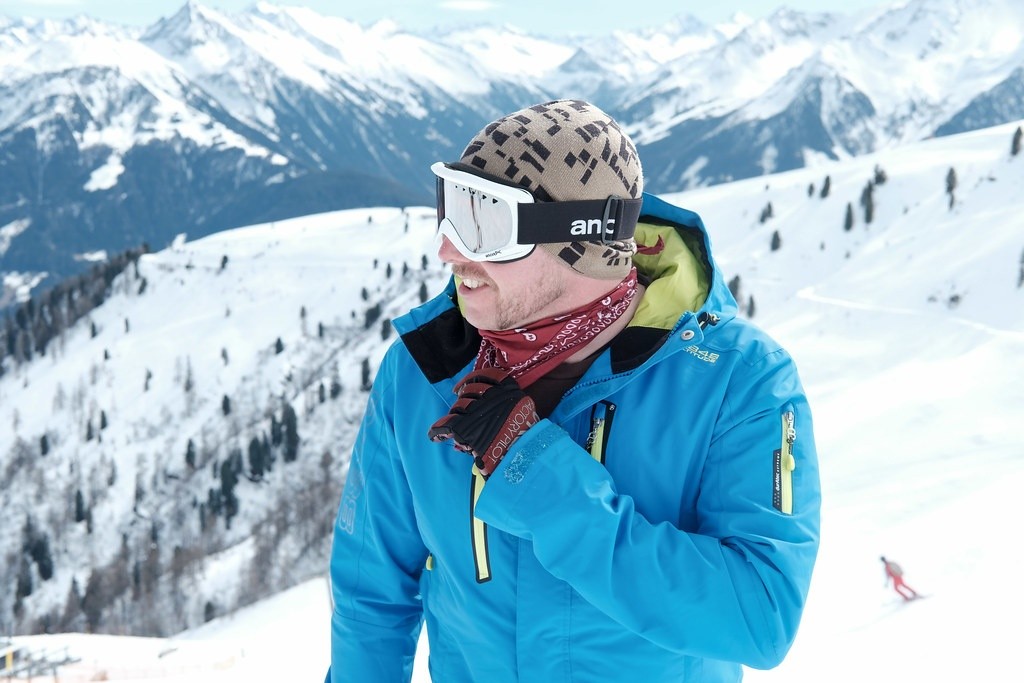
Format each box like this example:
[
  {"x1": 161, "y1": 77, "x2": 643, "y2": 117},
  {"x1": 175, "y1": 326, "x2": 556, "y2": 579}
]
[{"x1": 427, "y1": 367, "x2": 538, "y2": 486}]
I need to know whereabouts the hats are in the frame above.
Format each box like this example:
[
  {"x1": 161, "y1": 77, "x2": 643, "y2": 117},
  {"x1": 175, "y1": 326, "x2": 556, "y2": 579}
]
[{"x1": 461, "y1": 97, "x2": 644, "y2": 280}]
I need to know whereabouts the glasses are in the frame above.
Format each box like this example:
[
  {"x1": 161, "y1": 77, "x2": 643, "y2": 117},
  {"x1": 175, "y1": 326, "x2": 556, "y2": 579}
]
[{"x1": 431, "y1": 161, "x2": 539, "y2": 265}]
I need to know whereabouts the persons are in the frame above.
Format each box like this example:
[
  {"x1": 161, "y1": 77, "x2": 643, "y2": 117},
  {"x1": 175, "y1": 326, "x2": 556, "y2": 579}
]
[
  {"x1": 880, "y1": 556, "x2": 917, "y2": 602},
  {"x1": 323, "y1": 100, "x2": 822, "y2": 683}
]
